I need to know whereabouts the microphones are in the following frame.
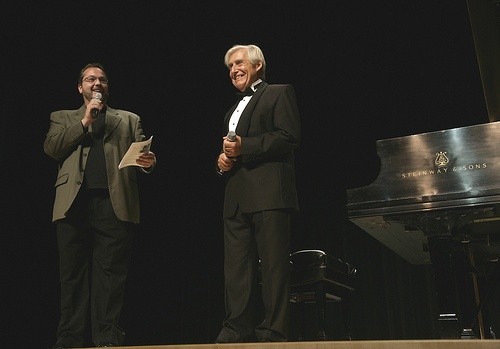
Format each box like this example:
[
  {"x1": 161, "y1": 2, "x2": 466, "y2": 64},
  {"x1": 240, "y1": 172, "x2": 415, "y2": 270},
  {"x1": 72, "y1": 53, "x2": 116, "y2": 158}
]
[
  {"x1": 92, "y1": 92, "x2": 103, "y2": 118},
  {"x1": 219, "y1": 131, "x2": 237, "y2": 175}
]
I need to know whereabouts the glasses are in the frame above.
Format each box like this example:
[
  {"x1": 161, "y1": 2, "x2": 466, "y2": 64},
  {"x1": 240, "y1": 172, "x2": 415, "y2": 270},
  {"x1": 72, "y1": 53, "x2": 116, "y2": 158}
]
[{"x1": 81, "y1": 76, "x2": 108, "y2": 84}]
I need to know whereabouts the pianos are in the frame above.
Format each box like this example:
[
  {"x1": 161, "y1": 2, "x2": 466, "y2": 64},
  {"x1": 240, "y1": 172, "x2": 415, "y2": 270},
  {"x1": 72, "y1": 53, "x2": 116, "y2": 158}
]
[{"x1": 345, "y1": 119, "x2": 500, "y2": 342}]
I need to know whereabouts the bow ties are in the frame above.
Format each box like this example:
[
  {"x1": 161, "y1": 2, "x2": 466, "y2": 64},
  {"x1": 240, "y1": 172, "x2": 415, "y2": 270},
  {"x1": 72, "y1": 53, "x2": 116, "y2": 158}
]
[{"x1": 237, "y1": 87, "x2": 255, "y2": 101}]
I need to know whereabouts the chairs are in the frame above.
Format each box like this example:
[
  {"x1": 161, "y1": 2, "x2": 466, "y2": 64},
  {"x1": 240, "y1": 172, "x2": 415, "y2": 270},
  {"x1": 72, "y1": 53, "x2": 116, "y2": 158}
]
[{"x1": 289, "y1": 249, "x2": 353, "y2": 340}]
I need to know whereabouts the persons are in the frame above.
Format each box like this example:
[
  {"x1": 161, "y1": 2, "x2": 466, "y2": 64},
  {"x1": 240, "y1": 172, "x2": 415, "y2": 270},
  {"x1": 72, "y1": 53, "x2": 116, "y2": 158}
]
[
  {"x1": 212, "y1": 44, "x2": 302, "y2": 345},
  {"x1": 42, "y1": 62, "x2": 156, "y2": 349}
]
